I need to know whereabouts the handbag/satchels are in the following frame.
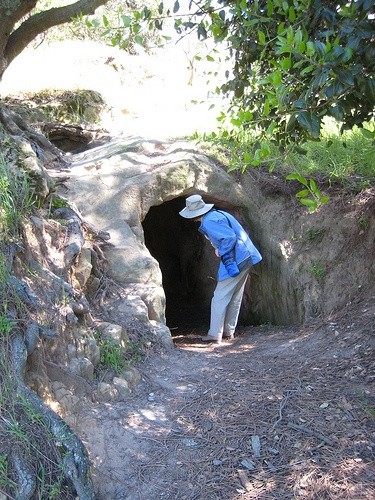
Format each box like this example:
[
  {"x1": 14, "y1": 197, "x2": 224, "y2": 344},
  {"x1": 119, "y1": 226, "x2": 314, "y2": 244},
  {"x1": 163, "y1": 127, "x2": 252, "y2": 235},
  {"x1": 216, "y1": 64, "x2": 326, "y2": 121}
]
[{"x1": 211, "y1": 211, "x2": 240, "y2": 278}]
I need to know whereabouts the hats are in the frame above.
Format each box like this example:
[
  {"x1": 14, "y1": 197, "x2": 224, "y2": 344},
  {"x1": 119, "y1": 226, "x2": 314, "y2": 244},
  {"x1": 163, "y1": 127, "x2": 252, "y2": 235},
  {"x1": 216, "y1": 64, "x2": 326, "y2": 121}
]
[{"x1": 179, "y1": 195, "x2": 214, "y2": 219}]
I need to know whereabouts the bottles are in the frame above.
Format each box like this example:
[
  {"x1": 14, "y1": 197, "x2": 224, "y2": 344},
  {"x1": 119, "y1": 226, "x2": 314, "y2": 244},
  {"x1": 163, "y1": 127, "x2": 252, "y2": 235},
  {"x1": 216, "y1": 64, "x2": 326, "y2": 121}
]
[{"x1": 221, "y1": 253, "x2": 239, "y2": 277}]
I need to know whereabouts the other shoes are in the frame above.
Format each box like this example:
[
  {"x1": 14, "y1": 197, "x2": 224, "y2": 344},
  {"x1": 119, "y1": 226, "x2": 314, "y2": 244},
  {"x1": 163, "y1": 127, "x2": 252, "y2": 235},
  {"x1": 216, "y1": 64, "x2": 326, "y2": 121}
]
[
  {"x1": 202, "y1": 336, "x2": 222, "y2": 344},
  {"x1": 223, "y1": 334, "x2": 235, "y2": 340}
]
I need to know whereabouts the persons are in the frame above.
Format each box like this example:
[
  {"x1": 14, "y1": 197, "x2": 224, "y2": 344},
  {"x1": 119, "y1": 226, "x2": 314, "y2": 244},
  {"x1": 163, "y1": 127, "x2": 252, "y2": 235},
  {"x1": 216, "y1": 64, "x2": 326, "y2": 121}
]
[{"x1": 179, "y1": 195, "x2": 263, "y2": 341}]
